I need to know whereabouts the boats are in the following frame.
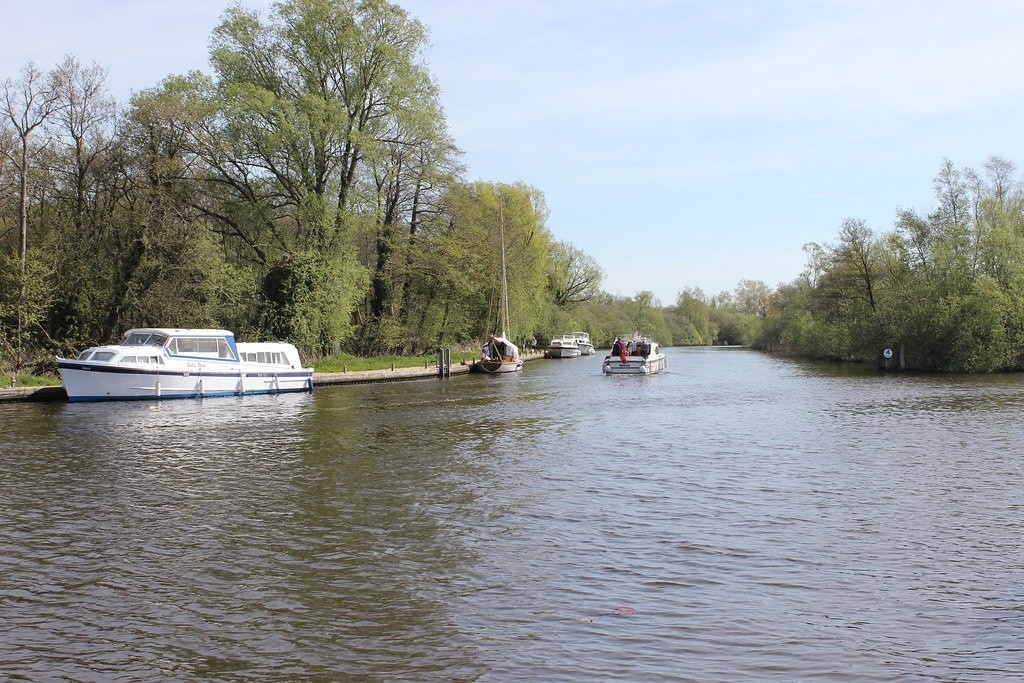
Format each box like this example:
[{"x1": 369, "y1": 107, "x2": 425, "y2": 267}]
[
  {"x1": 547, "y1": 331, "x2": 595, "y2": 358},
  {"x1": 602, "y1": 332, "x2": 668, "y2": 375},
  {"x1": 55, "y1": 327, "x2": 314, "y2": 401}
]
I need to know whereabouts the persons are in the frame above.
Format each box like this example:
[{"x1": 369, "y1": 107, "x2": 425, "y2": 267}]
[
  {"x1": 521, "y1": 335, "x2": 526, "y2": 352},
  {"x1": 613, "y1": 337, "x2": 648, "y2": 358},
  {"x1": 531, "y1": 338, "x2": 537, "y2": 350}
]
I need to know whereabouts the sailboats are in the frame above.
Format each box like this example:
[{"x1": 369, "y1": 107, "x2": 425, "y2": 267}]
[{"x1": 469, "y1": 196, "x2": 523, "y2": 374}]
